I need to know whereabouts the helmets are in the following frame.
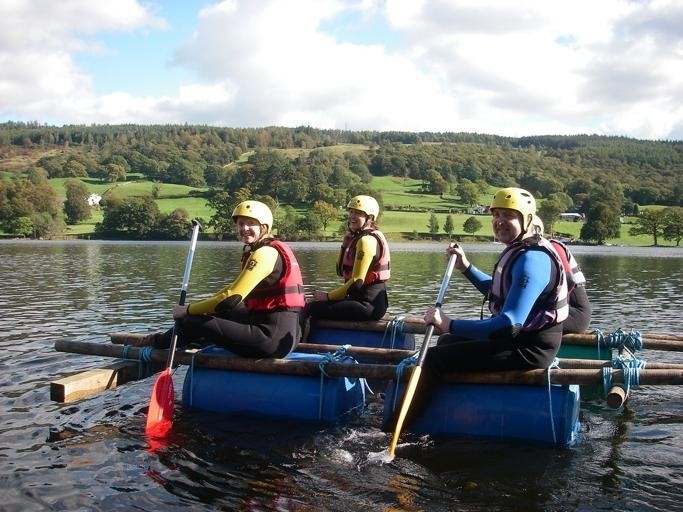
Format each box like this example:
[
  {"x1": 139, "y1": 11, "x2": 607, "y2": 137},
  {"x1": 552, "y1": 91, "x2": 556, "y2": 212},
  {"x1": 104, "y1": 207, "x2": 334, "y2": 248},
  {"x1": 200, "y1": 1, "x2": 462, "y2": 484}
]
[
  {"x1": 350, "y1": 215, "x2": 352, "y2": 216},
  {"x1": 488, "y1": 185, "x2": 537, "y2": 232},
  {"x1": 345, "y1": 192, "x2": 381, "y2": 222},
  {"x1": 531, "y1": 214, "x2": 544, "y2": 235},
  {"x1": 230, "y1": 200, "x2": 275, "y2": 235}
]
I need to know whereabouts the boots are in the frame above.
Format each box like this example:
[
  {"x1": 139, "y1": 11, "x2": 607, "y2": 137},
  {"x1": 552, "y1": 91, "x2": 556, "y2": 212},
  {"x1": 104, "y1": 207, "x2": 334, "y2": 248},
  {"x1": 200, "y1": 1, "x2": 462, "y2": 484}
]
[{"x1": 110, "y1": 329, "x2": 163, "y2": 350}]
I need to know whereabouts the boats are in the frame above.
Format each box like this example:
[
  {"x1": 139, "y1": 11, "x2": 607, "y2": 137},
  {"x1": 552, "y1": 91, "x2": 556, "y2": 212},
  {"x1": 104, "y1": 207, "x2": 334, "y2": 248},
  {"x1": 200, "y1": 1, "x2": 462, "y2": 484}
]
[{"x1": 49, "y1": 313, "x2": 682, "y2": 445}]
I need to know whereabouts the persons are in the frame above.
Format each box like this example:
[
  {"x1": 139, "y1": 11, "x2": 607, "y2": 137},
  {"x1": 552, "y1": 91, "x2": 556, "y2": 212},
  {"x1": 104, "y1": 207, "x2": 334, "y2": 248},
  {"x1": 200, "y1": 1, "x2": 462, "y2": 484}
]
[
  {"x1": 543, "y1": 235, "x2": 593, "y2": 335},
  {"x1": 395, "y1": 187, "x2": 570, "y2": 432},
  {"x1": 111, "y1": 201, "x2": 310, "y2": 357},
  {"x1": 298, "y1": 195, "x2": 389, "y2": 340}
]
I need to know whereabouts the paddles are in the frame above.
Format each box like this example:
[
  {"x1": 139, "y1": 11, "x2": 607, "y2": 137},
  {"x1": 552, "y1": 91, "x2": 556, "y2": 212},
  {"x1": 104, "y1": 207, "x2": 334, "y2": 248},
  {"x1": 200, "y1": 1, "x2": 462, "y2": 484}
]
[
  {"x1": 389, "y1": 252, "x2": 457, "y2": 456},
  {"x1": 145, "y1": 225, "x2": 199, "y2": 438}
]
